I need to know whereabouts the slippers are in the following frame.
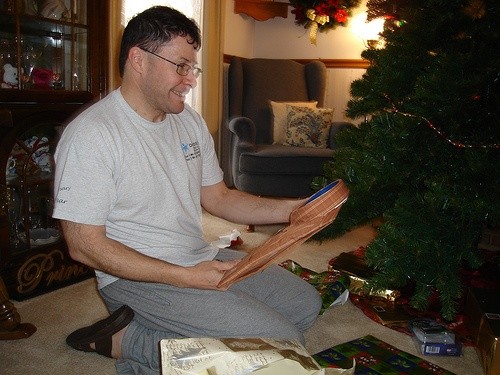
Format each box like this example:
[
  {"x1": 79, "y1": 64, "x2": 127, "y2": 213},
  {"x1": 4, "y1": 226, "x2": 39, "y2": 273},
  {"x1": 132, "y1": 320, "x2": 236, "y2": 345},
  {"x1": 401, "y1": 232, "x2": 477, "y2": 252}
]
[{"x1": 66, "y1": 304, "x2": 135, "y2": 359}]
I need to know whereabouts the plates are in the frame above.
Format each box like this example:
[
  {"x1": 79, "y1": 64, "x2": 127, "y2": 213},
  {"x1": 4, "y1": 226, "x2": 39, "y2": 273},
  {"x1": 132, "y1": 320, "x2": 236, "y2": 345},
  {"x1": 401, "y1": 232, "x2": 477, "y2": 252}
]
[
  {"x1": 5, "y1": 173, "x2": 18, "y2": 181},
  {"x1": 17, "y1": 228, "x2": 60, "y2": 246}
]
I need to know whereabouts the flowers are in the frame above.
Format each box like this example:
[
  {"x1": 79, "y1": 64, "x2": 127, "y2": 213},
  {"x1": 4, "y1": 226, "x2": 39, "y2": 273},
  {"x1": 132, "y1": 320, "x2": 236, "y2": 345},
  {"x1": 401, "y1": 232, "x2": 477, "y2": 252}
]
[{"x1": 287, "y1": 0, "x2": 364, "y2": 47}]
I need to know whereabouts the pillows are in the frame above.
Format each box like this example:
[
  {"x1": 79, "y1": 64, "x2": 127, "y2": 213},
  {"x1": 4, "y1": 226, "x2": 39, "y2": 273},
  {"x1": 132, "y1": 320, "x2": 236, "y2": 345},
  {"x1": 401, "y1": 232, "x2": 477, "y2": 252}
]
[
  {"x1": 282, "y1": 103, "x2": 335, "y2": 150},
  {"x1": 269, "y1": 99, "x2": 318, "y2": 145}
]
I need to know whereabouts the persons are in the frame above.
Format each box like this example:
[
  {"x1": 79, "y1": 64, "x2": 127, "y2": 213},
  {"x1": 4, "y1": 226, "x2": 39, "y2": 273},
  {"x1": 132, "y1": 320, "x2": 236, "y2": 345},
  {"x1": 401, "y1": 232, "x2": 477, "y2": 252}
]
[{"x1": 52, "y1": 6, "x2": 323, "y2": 375}]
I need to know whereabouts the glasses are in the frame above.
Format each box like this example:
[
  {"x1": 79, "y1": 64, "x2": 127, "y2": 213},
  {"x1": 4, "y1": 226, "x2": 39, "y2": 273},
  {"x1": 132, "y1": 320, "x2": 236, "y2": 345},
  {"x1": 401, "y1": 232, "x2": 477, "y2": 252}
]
[{"x1": 138, "y1": 48, "x2": 204, "y2": 79}]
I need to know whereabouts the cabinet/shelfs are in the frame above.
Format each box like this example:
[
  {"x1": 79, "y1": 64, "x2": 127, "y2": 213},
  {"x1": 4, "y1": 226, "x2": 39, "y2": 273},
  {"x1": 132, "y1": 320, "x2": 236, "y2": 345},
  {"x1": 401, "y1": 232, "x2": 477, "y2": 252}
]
[{"x1": 0, "y1": 1, "x2": 110, "y2": 299}]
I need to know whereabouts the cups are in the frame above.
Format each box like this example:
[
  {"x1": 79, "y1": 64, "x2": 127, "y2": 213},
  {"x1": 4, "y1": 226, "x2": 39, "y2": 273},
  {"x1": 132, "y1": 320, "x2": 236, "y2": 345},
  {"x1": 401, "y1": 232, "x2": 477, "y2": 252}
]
[{"x1": 5, "y1": 156, "x2": 15, "y2": 176}]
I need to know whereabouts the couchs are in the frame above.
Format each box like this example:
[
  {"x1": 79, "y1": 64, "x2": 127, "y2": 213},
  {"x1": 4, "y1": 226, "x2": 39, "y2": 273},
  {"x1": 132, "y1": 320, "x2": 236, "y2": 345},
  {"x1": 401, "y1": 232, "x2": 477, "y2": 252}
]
[{"x1": 227, "y1": 56, "x2": 357, "y2": 198}]
